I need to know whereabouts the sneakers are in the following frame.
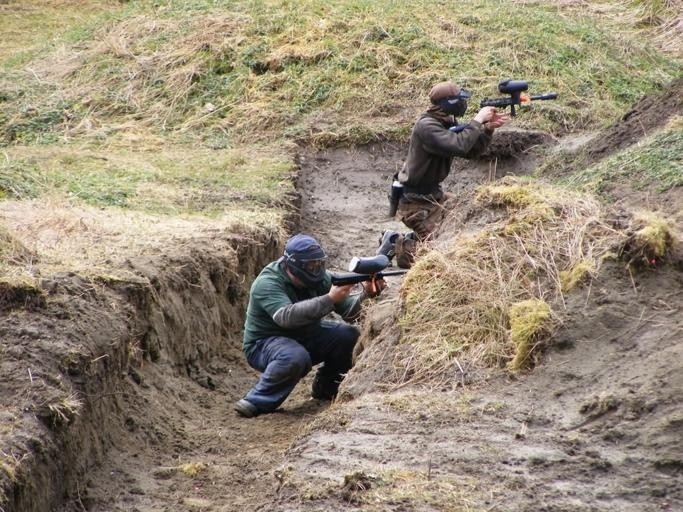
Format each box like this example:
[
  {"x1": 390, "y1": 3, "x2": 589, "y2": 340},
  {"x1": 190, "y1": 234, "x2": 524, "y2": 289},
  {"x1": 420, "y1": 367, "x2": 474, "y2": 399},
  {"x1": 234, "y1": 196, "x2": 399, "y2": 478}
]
[
  {"x1": 233, "y1": 399, "x2": 258, "y2": 418},
  {"x1": 311, "y1": 371, "x2": 345, "y2": 400},
  {"x1": 376, "y1": 229, "x2": 415, "y2": 268}
]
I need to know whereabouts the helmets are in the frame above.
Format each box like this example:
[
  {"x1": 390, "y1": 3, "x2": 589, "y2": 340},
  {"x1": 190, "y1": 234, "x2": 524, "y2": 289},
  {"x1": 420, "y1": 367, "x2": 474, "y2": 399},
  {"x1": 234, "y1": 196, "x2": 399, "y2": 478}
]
[
  {"x1": 283, "y1": 234, "x2": 328, "y2": 288},
  {"x1": 429, "y1": 82, "x2": 471, "y2": 116}
]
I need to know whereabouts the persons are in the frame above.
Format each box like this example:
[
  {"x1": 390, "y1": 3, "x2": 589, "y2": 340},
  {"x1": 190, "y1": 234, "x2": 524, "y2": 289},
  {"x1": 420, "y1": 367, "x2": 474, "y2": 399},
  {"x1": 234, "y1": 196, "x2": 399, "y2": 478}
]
[
  {"x1": 375, "y1": 81, "x2": 518, "y2": 270},
  {"x1": 230, "y1": 232, "x2": 388, "y2": 419}
]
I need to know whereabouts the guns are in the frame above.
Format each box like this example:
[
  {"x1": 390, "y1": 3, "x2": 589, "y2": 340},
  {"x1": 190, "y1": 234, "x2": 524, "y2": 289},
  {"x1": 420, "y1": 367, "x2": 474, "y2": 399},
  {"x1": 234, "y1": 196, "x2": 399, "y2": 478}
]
[
  {"x1": 481, "y1": 80, "x2": 559, "y2": 116},
  {"x1": 331, "y1": 255, "x2": 408, "y2": 286}
]
